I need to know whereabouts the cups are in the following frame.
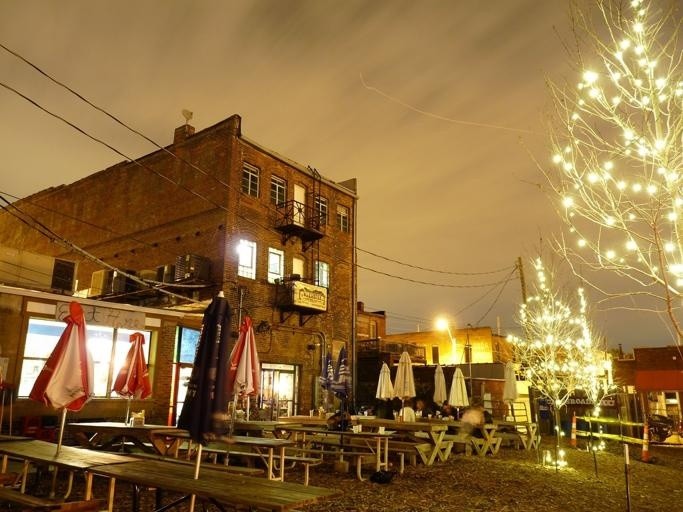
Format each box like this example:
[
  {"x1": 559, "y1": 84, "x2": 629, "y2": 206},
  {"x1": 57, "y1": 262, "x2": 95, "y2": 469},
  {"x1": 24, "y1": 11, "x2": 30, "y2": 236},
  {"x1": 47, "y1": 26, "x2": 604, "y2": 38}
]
[
  {"x1": 378, "y1": 426, "x2": 386, "y2": 435},
  {"x1": 352, "y1": 423, "x2": 363, "y2": 434}
]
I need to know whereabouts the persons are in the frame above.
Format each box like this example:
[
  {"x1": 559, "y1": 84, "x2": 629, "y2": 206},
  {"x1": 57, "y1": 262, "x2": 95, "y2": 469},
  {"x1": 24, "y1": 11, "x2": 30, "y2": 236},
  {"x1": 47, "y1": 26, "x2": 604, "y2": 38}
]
[
  {"x1": 399, "y1": 400, "x2": 416, "y2": 422},
  {"x1": 414, "y1": 400, "x2": 431, "y2": 417},
  {"x1": 471, "y1": 404, "x2": 493, "y2": 438},
  {"x1": 439, "y1": 400, "x2": 457, "y2": 434}
]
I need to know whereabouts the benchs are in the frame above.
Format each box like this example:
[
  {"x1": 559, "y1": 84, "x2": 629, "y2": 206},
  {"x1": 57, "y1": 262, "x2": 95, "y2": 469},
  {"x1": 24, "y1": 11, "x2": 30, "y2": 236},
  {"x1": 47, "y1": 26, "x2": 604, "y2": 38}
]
[{"x1": 1, "y1": 488, "x2": 61, "y2": 508}]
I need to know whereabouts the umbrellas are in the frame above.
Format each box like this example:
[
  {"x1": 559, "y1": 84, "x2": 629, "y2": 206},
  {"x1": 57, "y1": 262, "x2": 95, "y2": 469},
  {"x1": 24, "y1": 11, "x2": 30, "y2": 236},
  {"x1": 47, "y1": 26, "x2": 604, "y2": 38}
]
[
  {"x1": 227, "y1": 315, "x2": 260, "y2": 438},
  {"x1": 29, "y1": 301, "x2": 95, "y2": 498},
  {"x1": 331, "y1": 346, "x2": 353, "y2": 448},
  {"x1": 448, "y1": 368, "x2": 470, "y2": 421},
  {"x1": 114, "y1": 333, "x2": 151, "y2": 448},
  {"x1": 318, "y1": 352, "x2": 334, "y2": 413},
  {"x1": 433, "y1": 363, "x2": 447, "y2": 415},
  {"x1": 177, "y1": 291, "x2": 231, "y2": 512},
  {"x1": 375, "y1": 360, "x2": 393, "y2": 418},
  {"x1": 393, "y1": 351, "x2": 416, "y2": 422}
]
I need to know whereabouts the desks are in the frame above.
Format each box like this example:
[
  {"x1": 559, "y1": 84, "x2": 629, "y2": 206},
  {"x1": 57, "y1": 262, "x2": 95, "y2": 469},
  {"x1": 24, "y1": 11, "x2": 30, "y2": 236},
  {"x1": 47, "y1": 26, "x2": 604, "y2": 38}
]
[
  {"x1": 2, "y1": 440, "x2": 142, "y2": 510},
  {"x1": 86, "y1": 460, "x2": 344, "y2": 510},
  {"x1": 70, "y1": 409, "x2": 500, "y2": 486}
]
